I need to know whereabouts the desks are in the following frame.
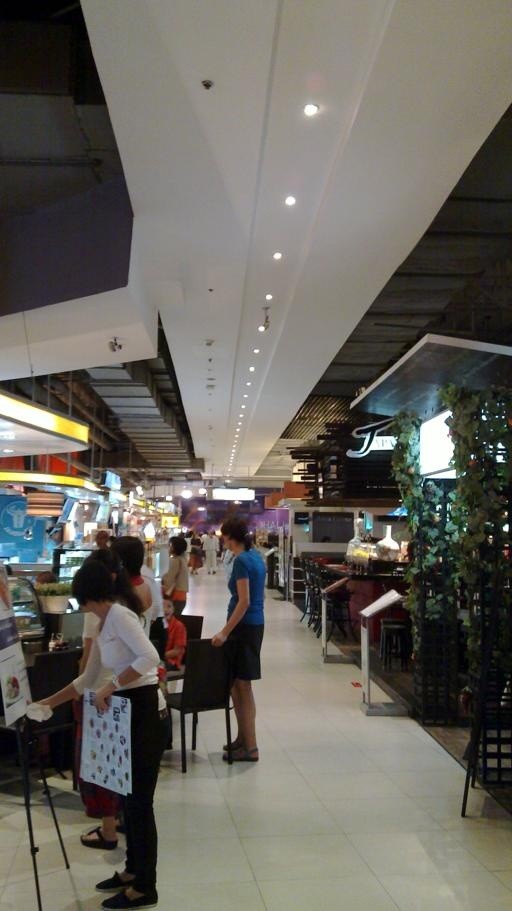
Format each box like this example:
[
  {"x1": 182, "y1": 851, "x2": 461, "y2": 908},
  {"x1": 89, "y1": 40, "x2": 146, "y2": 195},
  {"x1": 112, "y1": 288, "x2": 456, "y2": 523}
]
[{"x1": 0, "y1": 694, "x2": 76, "y2": 909}]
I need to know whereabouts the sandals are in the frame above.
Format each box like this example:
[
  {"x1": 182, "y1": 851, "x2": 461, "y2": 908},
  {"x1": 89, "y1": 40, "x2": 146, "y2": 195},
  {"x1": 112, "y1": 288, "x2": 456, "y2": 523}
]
[{"x1": 223, "y1": 740, "x2": 258, "y2": 761}]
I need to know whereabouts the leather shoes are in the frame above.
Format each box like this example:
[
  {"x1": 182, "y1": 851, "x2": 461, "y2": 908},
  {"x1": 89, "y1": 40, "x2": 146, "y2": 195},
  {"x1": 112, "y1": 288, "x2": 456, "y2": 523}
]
[
  {"x1": 96, "y1": 870, "x2": 137, "y2": 892},
  {"x1": 102, "y1": 890, "x2": 157, "y2": 909}
]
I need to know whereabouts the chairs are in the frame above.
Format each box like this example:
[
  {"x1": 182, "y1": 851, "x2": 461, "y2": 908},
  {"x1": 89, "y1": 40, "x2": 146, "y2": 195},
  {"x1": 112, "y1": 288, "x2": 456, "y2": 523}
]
[
  {"x1": 300, "y1": 555, "x2": 359, "y2": 644},
  {"x1": 378, "y1": 584, "x2": 411, "y2": 672},
  {"x1": 29, "y1": 616, "x2": 236, "y2": 788}
]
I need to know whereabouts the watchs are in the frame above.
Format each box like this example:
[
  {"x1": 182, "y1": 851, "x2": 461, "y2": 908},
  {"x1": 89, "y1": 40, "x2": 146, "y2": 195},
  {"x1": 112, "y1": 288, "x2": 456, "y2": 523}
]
[{"x1": 107, "y1": 671, "x2": 122, "y2": 698}]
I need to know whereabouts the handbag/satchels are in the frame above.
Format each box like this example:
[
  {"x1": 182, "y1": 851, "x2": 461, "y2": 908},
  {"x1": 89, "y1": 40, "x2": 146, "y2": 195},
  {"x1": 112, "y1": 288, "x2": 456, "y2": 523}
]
[{"x1": 164, "y1": 579, "x2": 175, "y2": 597}]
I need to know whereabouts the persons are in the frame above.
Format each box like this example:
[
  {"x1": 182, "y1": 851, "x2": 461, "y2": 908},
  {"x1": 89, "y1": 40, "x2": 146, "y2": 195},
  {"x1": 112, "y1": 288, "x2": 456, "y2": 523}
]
[
  {"x1": 210, "y1": 517, "x2": 268, "y2": 765},
  {"x1": 109, "y1": 535, "x2": 246, "y2": 672},
  {"x1": 42, "y1": 525, "x2": 61, "y2": 555},
  {"x1": 24, "y1": 559, "x2": 173, "y2": 911},
  {"x1": 73, "y1": 549, "x2": 129, "y2": 850},
  {"x1": 93, "y1": 531, "x2": 114, "y2": 557}
]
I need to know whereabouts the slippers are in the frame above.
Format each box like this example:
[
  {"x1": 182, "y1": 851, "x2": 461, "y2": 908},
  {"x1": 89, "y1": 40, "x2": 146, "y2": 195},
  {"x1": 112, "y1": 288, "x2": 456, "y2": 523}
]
[{"x1": 82, "y1": 827, "x2": 117, "y2": 850}]
